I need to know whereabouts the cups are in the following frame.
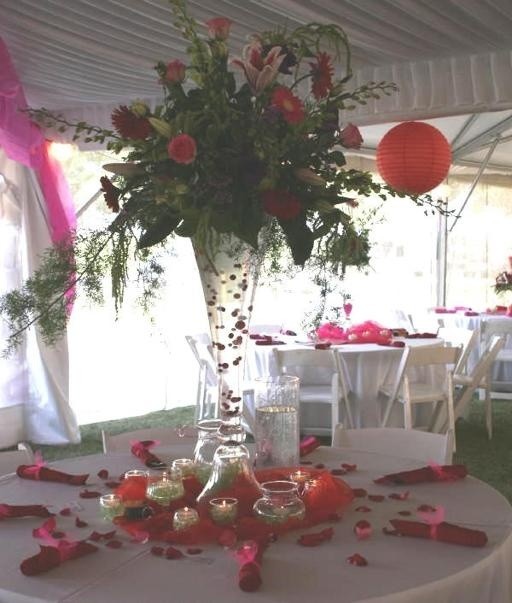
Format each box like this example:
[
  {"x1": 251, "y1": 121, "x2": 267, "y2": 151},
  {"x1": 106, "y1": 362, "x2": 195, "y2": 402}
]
[
  {"x1": 252, "y1": 374, "x2": 301, "y2": 471},
  {"x1": 99, "y1": 457, "x2": 237, "y2": 533}
]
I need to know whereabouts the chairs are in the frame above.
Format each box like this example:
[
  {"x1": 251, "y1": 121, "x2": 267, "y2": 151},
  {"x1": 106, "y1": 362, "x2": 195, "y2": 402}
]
[
  {"x1": 185, "y1": 323, "x2": 464, "y2": 453},
  {"x1": 100, "y1": 426, "x2": 179, "y2": 453},
  {"x1": 1, "y1": 442, "x2": 35, "y2": 476},
  {"x1": 394, "y1": 306, "x2": 511, "y2": 401},
  {"x1": 332, "y1": 423, "x2": 454, "y2": 466}
]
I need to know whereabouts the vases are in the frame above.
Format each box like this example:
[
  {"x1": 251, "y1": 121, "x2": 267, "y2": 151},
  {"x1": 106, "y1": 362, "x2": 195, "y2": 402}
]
[{"x1": 193, "y1": 220, "x2": 269, "y2": 521}]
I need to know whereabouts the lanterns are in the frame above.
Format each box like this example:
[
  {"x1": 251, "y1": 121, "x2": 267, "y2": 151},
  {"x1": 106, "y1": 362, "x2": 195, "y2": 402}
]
[{"x1": 376, "y1": 120, "x2": 454, "y2": 196}]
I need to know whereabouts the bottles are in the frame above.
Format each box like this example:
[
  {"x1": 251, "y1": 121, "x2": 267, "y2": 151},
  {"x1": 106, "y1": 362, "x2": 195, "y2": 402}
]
[
  {"x1": 253, "y1": 481, "x2": 307, "y2": 526},
  {"x1": 195, "y1": 420, "x2": 225, "y2": 474}
]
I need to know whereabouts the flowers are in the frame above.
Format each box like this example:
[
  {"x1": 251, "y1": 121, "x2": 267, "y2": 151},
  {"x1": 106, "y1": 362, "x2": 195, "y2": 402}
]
[{"x1": 100, "y1": 0, "x2": 362, "y2": 244}]
